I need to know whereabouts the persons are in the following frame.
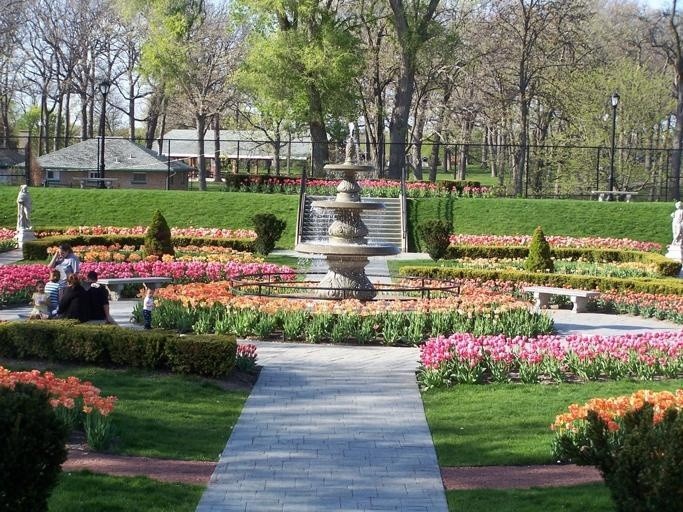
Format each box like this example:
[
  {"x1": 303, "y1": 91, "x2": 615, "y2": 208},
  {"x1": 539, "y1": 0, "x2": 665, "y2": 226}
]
[
  {"x1": 670, "y1": 202, "x2": 683, "y2": 245},
  {"x1": 48, "y1": 244, "x2": 80, "y2": 286},
  {"x1": 44, "y1": 271, "x2": 63, "y2": 319},
  {"x1": 142, "y1": 282, "x2": 155, "y2": 330},
  {"x1": 17, "y1": 185, "x2": 31, "y2": 229},
  {"x1": 57, "y1": 274, "x2": 87, "y2": 319},
  {"x1": 30, "y1": 280, "x2": 51, "y2": 320},
  {"x1": 82, "y1": 271, "x2": 117, "y2": 324}
]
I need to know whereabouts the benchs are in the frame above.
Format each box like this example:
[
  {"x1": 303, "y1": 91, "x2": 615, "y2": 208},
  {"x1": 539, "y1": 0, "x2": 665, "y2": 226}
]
[
  {"x1": 591, "y1": 191, "x2": 638, "y2": 203},
  {"x1": 97, "y1": 277, "x2": 172, "y2": 301},
  {"x1": 73, "y1": 177, "x2": 119, "y2": 189},
  {"x1": 524, "y1": 286, "x2": 600, "y2": 313}
]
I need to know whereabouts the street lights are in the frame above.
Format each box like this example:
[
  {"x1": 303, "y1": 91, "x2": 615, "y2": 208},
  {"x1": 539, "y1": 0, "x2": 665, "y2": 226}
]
[
  {"x1": 97, "y1": 81, "x2": 110, "y2": 190},
  {"x1": 605, "y1": 92, "x2": 619, "y2": 202}
]
